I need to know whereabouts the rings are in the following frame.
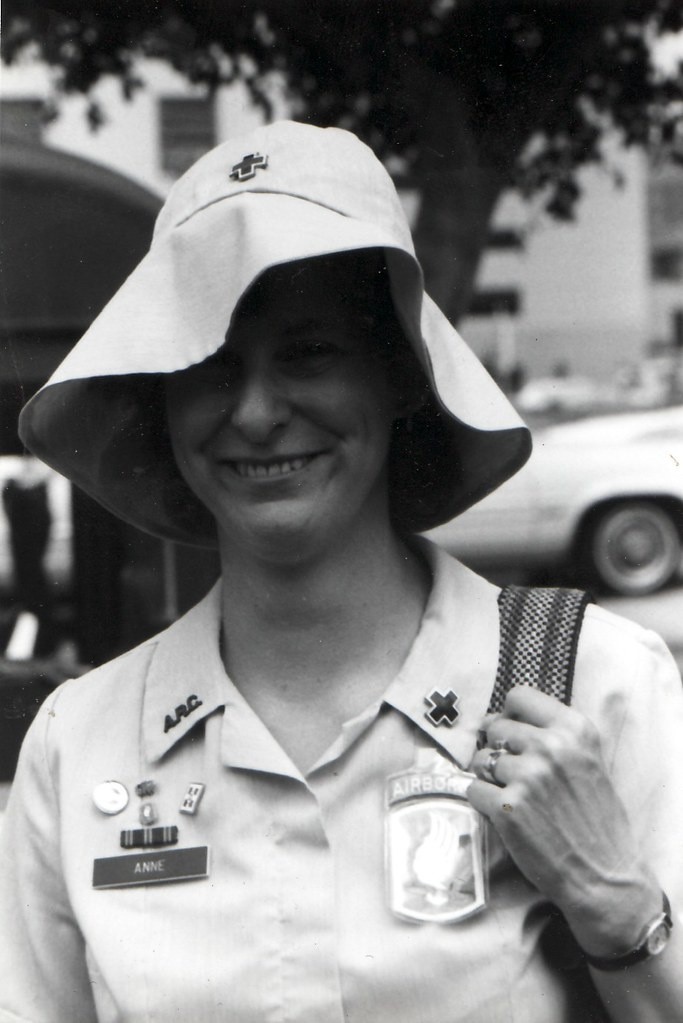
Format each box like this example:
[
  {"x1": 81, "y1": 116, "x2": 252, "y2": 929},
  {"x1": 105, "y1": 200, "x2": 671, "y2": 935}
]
[{"x1": 484, "y1": 739, "x2": 512, "y2": 786}]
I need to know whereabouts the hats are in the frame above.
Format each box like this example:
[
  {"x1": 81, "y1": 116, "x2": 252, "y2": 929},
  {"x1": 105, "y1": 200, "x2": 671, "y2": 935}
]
[{"x1": 16, "y1": 118, "x2": 533, "y2": 551}]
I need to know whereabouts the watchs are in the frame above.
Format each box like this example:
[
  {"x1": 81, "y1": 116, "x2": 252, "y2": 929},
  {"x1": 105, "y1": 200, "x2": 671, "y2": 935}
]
[{"x1": 579, "y1": 882, "x2": 676, "y2": 973}]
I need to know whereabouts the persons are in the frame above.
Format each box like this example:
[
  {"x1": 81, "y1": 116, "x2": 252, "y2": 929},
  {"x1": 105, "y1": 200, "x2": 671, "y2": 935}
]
[{"x1": 0, "y1": 121, "x2": 683, "y2": 1022}]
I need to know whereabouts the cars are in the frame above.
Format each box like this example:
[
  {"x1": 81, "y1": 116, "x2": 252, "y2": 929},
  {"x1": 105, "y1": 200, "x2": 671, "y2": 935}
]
[{"x1": 426, "y1": 404, "x2": 683, "y2": 601}]
